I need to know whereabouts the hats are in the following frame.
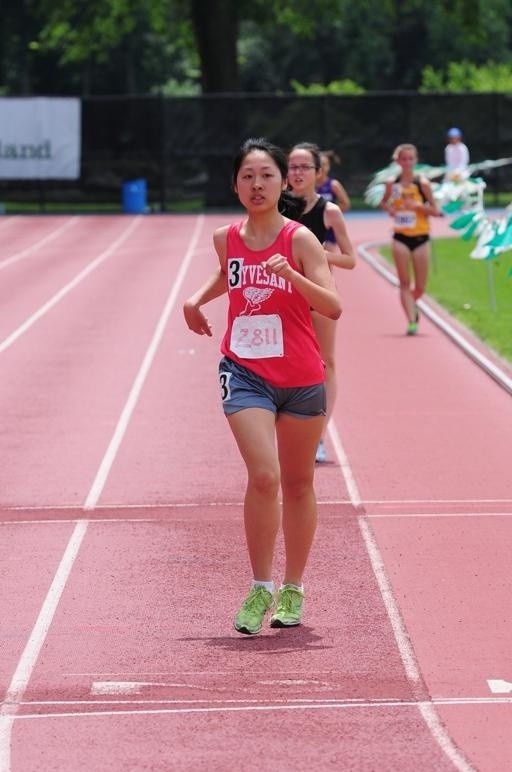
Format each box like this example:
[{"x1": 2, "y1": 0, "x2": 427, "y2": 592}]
[{"x1": 447, "y1": 127, "x2": 462, "y2": 136}]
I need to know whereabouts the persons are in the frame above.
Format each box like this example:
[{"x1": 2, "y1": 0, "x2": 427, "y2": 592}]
[
  {"x1": 382, "y1": 142, "x2": 445, "y2": 335},
  {"x1": 284, "y1": 141, "x2": 357, "y2": 463},
  {"x1": 317, "y1": 148, "x2": 351, "y2": 274},
  {"x1": 444, "y1": 127, "x2": 470, "y2": 181},
  {"x1": 183, "y1": 137, "x2": 342, "y2": 635}
]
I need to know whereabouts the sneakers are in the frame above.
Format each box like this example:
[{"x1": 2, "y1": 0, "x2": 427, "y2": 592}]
[
  {"x1": 235, "y1": 584, "x2": 275, "y2": 633},
  {"x1": 407, "y1": 304, "x2": 419, "y2": 335},
  {"x1": 315, "y1": 443, "x2": 326, "y2": 462},
  {"x1": 271, "y1": 584, "x2": 303, "y2": 626}
]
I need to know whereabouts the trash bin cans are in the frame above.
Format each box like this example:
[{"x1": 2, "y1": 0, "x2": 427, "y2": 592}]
[{"x1": 123, "y1": 179, "x2": 146, "y2": 213}]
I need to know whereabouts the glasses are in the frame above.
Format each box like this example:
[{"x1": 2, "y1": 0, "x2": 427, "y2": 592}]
[{"x1": 288, "y1": 165, "x2": 316, "y2": 172}]
